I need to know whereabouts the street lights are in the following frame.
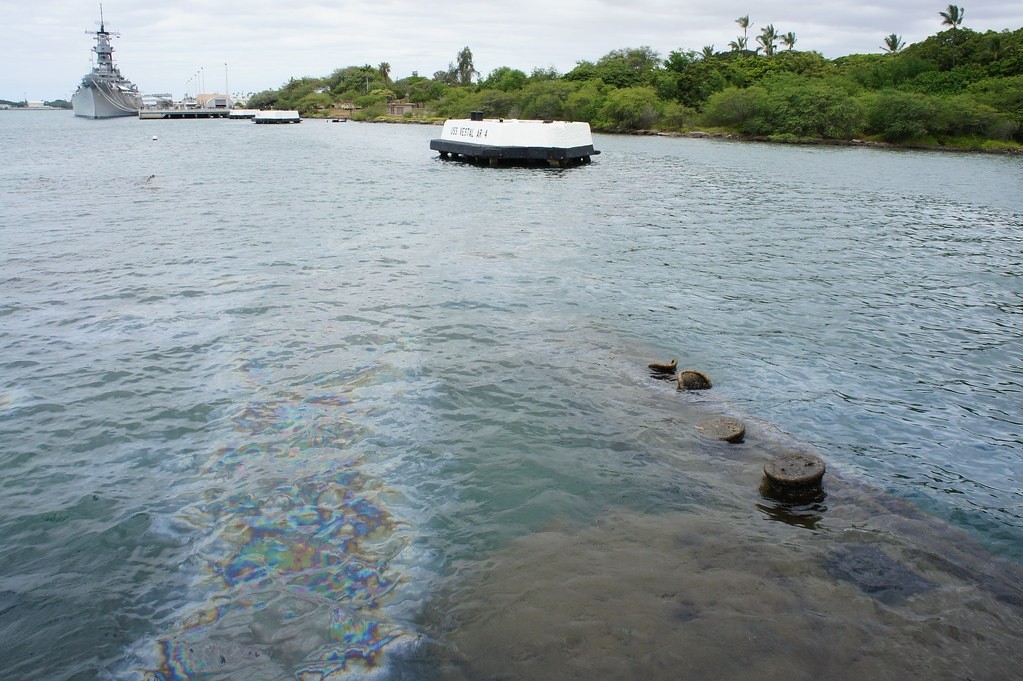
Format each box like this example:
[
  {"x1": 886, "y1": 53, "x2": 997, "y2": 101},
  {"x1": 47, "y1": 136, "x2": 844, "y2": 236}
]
[
  {"x1": 225, "y1": 62, "x2": 228, "y2": 97},
  {"x1": 185, "y1": 67, "x2": 206, "y2": 108}
]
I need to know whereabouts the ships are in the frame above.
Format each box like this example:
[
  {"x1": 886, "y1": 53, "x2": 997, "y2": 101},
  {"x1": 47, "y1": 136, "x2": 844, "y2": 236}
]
[{"x1": 70, "y1": 2, "x2": 144, "y2": 119}]
